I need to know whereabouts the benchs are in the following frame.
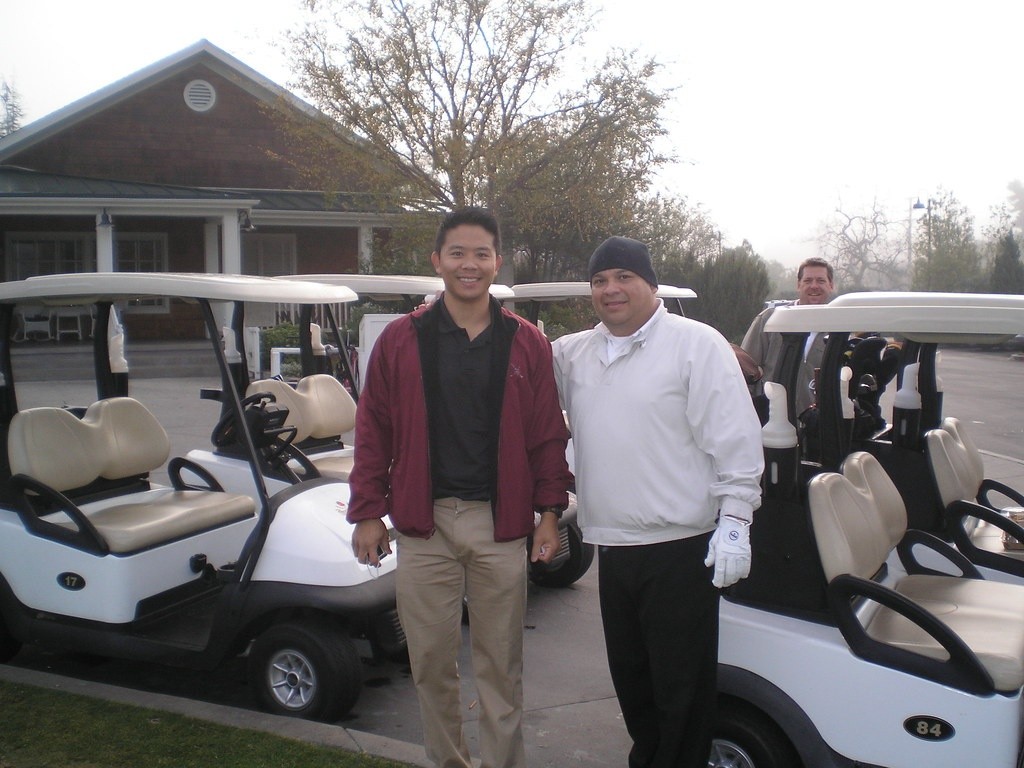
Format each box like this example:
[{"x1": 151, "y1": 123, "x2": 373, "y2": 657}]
[
  {"x1": 805, "y1": 450, "x2": 1024, "y2": 691},
  {"x1": 8, "y1": 397, "x2": 257, "y2": 558},
  {"x1": 245, "y1": 374, "x2": 359, "y2": 481},
  {"x1": 924, "y1": 417, "x2": 1024, "y2": 577}
]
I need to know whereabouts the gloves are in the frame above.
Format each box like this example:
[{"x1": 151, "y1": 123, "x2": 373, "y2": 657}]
[{"x1": 704, "y1": 515, "x2": 751, "y2": 588}]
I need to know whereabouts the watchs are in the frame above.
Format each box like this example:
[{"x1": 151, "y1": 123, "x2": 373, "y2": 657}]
[{"x1": 538, "y1": 506, "x2": 563, "y2": 519}]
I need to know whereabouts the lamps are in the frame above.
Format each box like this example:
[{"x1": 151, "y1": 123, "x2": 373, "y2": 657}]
[
  {"x1": 96, "y1": 208, "x2": 116, "y2": 229},
  {"x1": 238, "y1": 211, "x2": 257, "y2": 232}
]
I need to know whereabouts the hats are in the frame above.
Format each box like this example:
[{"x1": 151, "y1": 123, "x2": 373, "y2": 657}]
[{"x1": 589, "y1": 235, "x2": 658, "y2": 289}]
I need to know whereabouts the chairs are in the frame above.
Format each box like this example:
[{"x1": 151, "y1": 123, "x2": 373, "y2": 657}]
[
  {"x1": 10, "y1": 307, "x2": 54, "y2": 343},
  {"x1": 57, "y1": 311, "x2": 84, "y2": 342},
  {"x1": 88, "y1": 304, "x2": 122, "y2": 339}
]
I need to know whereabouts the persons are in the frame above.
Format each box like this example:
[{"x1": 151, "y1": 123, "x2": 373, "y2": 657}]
[
  {"x1": 419, "y1": 236, "x2": 764, "y2": 768},
  {"x1": 740, "y1": 257, "x2": 833, "y2": 456},
  {"x1": 344, "y1": 207, "x2": 571, "y2": 768}
]
